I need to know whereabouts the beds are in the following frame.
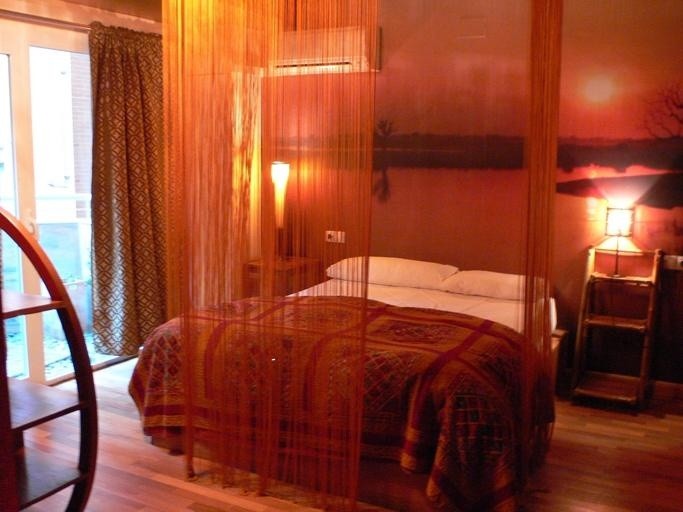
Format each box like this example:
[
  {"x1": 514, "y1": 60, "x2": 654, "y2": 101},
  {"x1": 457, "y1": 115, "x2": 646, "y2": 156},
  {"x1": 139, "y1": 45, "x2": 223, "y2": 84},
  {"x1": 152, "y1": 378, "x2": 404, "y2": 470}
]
[{"x1": 128, "y1": 280, "x2": 570, "y2": 512}]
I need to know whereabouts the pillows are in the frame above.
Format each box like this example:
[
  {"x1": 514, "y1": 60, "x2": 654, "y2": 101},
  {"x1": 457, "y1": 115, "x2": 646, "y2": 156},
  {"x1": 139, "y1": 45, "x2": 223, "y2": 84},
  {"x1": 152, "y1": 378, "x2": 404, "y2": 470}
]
[
  {"x1": 439, "y1": 270, "x2": 550, "y2": 303},
  {"x1": 326, "y1": 256, "x2": 458, "y2": 289}
]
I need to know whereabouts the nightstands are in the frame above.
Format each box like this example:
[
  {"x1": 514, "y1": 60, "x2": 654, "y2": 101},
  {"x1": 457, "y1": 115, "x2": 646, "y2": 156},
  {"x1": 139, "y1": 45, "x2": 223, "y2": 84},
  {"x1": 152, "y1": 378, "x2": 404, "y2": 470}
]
[{"x1": 241, "y1": 254, "x2": 326, "y2": 300}]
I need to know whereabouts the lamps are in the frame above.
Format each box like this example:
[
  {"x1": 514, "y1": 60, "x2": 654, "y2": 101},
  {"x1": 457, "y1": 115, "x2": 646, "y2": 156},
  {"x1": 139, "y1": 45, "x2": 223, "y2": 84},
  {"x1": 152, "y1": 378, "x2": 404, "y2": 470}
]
[
  {"x1": 594, "y1": 204, "x2": 643, "y2": 277},
  {"x1": 270, "y1": 160, "x2": 290, "y2": 261}
]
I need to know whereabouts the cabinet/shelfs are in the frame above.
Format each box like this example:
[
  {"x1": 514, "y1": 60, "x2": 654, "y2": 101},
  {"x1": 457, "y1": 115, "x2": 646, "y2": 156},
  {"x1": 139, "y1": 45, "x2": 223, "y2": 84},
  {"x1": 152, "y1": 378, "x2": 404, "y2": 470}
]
[
  {"x1": 0, "y1": 207, "x2": 98, "y2": 512},
  {"x1": 566, "y1": 245, "x2": 660, "y2": 411}
]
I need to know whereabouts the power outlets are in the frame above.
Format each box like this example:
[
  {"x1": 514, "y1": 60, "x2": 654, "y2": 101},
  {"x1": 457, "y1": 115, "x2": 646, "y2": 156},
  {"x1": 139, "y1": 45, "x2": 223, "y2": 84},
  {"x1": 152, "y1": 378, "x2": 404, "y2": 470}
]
[{"x1": 324, "y1": 230, "x2": 344, "y2": 244}]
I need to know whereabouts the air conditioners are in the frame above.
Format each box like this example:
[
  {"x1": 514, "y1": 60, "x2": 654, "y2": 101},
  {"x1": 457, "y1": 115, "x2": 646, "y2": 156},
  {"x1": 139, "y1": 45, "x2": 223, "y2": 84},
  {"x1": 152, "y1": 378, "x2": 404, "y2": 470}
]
[{"x1": 265, "y1": 22, "x2": 381, "y2": 77}]
